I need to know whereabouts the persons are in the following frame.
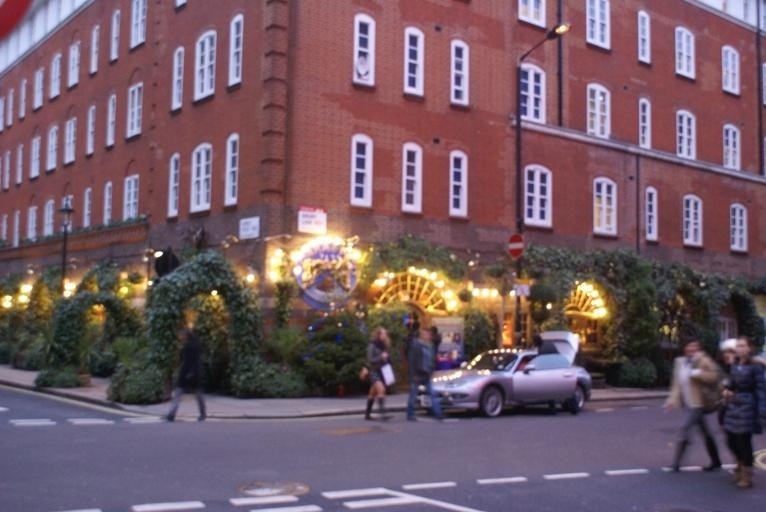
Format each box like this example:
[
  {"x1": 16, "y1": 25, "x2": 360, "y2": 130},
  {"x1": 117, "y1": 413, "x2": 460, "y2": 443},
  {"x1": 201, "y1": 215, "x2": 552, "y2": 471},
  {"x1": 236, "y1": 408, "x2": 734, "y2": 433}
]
[
  {"x1": 359, "y1": 327, "x2": 445, "y2": 421},
  {"x1": 663, "y1": 328, "x2": 766, "y2": 487},
  {"x1": 160, "y1": 328, "x2": 208, "y2": 421}
]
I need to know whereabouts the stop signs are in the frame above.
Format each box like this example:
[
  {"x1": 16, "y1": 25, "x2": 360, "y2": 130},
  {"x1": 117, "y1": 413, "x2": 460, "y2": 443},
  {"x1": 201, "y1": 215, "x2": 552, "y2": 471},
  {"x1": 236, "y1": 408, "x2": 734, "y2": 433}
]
[{"x1": 506, "y1": 233, "x2": 526, "y2": 262}]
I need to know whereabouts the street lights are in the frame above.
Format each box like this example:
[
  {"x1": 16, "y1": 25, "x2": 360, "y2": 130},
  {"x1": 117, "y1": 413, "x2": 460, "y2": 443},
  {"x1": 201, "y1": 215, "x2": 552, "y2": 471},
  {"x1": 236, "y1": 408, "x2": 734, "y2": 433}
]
[
  {"x1": 516, "y1": 20, "x2": 572, "y2": 350},
  {"x1": 57, "y1": 194, "x2": 74, "y2": 290}
]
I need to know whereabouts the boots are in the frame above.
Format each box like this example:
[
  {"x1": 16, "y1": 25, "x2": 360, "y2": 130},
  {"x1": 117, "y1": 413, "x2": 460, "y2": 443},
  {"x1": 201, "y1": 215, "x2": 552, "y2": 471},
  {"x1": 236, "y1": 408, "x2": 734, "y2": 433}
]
[
  {"x1": 661, "y1": 438, "x2": 689, "y2": 472},
  {"x1": 702, "y1": 441, "x2": 722, "y2": 470},
  {"x1": 364, "y1": 397, "x2": 391, "y2": 421},
  {"x1": 730, "y1": 462, "x2": 751, "y2": 490}
]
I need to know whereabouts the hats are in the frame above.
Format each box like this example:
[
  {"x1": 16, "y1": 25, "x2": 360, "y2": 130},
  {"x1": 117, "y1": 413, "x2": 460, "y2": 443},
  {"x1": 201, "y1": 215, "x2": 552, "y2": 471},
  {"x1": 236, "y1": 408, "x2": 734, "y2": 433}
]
[{"x1": 720, "y1": 339, "x2": 736, "y2": 351}]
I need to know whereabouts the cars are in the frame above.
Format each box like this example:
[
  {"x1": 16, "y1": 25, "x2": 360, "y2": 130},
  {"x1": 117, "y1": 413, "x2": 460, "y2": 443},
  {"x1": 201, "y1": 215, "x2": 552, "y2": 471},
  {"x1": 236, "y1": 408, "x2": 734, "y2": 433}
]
[{"x1": 417, "y1": 331, "x2": 593, "y2": 417}]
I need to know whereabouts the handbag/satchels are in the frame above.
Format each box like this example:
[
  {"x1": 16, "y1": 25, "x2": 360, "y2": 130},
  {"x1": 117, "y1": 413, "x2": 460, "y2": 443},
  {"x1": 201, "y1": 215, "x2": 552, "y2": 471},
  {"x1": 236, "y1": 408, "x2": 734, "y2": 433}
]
[{"x1": 360, "y1": 367, "x2": 368, "y2": 381}]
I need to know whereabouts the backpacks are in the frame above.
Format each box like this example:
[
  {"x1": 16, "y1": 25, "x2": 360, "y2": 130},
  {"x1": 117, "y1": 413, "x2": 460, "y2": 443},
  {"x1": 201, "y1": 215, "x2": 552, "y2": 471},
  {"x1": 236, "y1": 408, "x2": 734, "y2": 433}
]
[{"x1": 689, "y1": 350, "x2": 729, "y2": 413}]
